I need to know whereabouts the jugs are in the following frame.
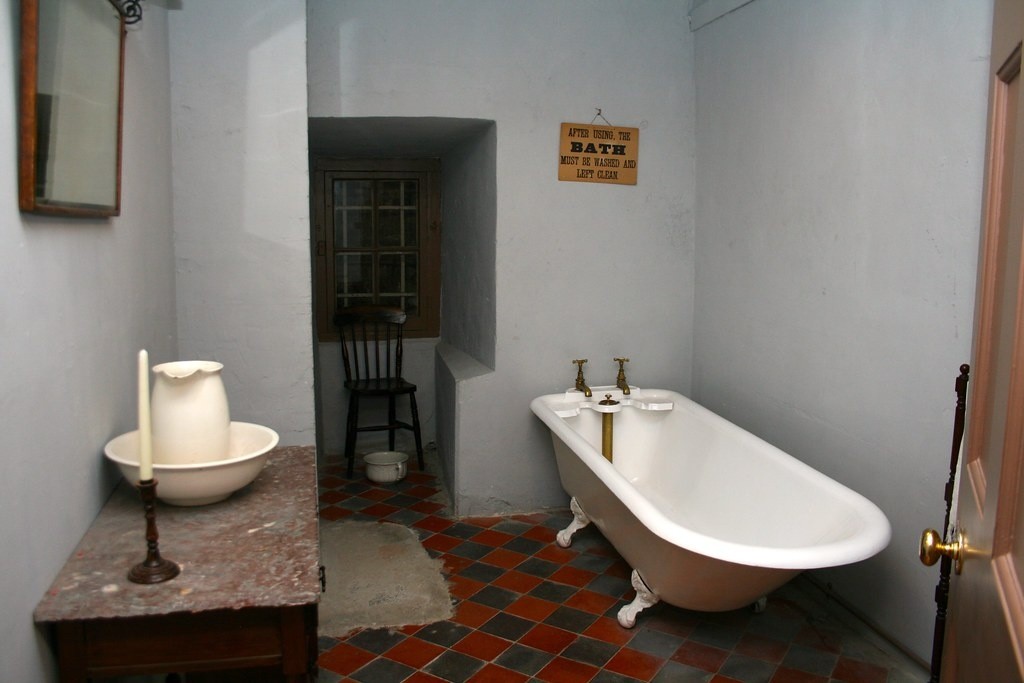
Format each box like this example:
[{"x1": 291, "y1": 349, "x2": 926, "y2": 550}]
[{"x1": 150, "y1": 360, "x2": 230, "y2": 464}]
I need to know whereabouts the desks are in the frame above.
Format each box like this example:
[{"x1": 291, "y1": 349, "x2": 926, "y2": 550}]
[{"x1": 32, "y1": 444, "x2": 323, "y2": 683}]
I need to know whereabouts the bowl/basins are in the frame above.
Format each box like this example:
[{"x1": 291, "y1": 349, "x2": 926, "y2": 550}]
[
  {"x1": 364, "y1": 452, "x2": 408, "y2": 484},
  {"x1": 104, "y1": 421, "x2": 280, "y2": 507}
]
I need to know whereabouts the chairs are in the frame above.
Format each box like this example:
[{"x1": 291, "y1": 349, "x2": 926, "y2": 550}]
[{"x1": 333, "y1": 306, "x2": 426, "y2": 478}]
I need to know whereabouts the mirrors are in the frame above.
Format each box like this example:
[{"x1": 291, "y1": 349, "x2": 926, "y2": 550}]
[{"x1": 17, "y1": 0, "x2": 126, "y2": 217}]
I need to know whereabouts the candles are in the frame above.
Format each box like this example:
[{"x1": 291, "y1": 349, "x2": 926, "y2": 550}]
[{"x1": 137, "y1": 348, "x2": 154, "y2": 481}]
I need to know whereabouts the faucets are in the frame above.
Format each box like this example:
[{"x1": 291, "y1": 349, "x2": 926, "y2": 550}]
[
  {"x1": 612, "y1": 356, "x2": 632, "y2": 395},
  {"x1": 572, "y1": 358, "x2": 593, "y2": 398}
]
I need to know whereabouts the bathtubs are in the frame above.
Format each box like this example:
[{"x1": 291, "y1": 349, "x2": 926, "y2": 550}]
[{"x1": 529, "y1": 385, "x2": 894, "y2": 615}]
